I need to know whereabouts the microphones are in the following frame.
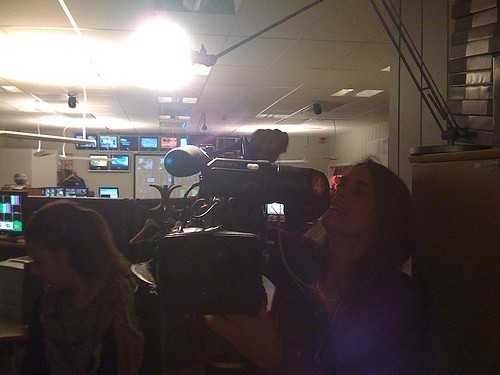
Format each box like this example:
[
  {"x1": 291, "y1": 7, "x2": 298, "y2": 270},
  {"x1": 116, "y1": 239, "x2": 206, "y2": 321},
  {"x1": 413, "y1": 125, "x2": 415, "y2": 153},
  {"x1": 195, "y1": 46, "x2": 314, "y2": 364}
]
[{"x1": 165, "y1": 146, "x2": 210, "y2": 177}]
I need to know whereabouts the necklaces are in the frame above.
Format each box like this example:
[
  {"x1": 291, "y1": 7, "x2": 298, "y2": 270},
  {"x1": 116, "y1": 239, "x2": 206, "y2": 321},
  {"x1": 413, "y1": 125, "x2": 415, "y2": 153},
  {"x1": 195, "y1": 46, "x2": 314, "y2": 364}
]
[{"x1": 313, "y1": 280, "x2": 347, "y2": 302}]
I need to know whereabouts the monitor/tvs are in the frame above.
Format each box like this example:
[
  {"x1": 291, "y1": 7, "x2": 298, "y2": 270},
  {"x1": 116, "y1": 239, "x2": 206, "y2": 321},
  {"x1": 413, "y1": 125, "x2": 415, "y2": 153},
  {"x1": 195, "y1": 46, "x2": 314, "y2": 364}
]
[
  {"x1": 31, "y1": 187, "x2": 88, "y2": 197},
  {"x1": 0, "y1": 189, "x2": 28, "y2": 241},
  {"x1": 98, "y1": 187, "x2": 119, "y2": 199},
  {"x1": 74, "y1": 132, "x2": 243, "y2": 169}
]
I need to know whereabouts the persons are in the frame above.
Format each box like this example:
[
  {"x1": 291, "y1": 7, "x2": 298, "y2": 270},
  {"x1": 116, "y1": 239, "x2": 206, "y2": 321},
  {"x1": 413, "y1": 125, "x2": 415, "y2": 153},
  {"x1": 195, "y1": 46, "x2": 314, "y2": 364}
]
[
  {"x1": 25, "y1": 199, "x2": 147, "y2": 375},
  {"x1": 202, "y1": 128, "x2": 437, "y2": 374}
]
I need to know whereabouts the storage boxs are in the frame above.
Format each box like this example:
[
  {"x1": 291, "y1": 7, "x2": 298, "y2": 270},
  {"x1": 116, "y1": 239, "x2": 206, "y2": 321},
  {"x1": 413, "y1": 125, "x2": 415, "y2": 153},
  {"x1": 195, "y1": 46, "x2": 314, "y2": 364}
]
[{"x1": 0, "y1": 256, "x2": 37, "y2": 324}]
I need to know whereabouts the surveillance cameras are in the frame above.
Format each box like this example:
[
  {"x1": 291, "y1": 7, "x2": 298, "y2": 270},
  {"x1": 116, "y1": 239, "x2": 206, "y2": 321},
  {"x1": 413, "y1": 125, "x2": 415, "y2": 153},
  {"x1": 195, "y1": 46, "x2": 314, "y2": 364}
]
[
  {"x1": 69, "y1": 94, "x2": 78, "y2": 108},
  {"x1": 312, "y1": 102, "x2": 322, "y2": 117}
]
